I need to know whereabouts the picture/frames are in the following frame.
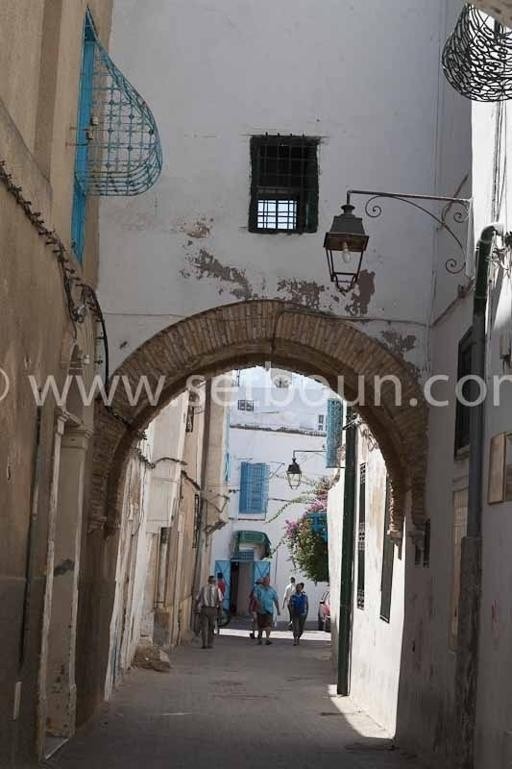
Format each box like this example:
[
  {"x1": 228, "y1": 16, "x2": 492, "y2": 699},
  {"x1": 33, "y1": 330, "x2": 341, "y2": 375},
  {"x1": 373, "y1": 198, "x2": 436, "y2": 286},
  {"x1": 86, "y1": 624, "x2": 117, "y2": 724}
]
[{"x1": 487, "y1": 431, "x2": 506, "y2": 505}]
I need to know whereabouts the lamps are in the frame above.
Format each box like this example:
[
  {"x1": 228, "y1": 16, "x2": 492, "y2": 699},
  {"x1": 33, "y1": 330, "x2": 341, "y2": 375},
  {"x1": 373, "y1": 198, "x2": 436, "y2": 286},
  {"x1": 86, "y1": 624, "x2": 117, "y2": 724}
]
[
  {"x1": 323, "y1": 190, "x2": 468, "y2": 295},
  {"x1": 285, "y1": 449, "x2": 330, "y2": 490}
]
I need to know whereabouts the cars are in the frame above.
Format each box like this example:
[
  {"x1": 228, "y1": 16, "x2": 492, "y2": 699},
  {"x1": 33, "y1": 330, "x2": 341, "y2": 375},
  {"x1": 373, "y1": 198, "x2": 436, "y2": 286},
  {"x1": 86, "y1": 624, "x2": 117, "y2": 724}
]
[{"x1": 318, "y1": 590, "x2": 330, "y2": 631}]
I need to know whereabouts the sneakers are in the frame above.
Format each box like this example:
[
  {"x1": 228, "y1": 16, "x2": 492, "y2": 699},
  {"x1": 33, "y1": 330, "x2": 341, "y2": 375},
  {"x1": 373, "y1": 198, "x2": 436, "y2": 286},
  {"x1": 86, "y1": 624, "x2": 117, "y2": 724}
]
[
  {"x1": 250, "y1": 633, "x2": 273, "y2": 645},
  {"x1": 293, "y1": 639, "x2": 301, "y2": 646}
]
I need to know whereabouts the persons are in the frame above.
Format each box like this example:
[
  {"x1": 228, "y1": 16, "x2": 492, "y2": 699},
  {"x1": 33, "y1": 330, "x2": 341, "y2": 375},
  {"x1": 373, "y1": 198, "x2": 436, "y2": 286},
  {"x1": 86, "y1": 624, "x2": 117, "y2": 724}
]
[
  {"x1": 196, "y1": 576, "x2": 224, "y2": 648},
  {"x1": 249, "y1": 577, "x2": 263, "y2": 639},
  {"x1": 253, "y1": 576, "x2": 280, "y2": 644},
  {"x1": 282, "y1": 577, "x2": 297, "y2": 630},
  {"x1": 288, "y1": 584, "x2": 309, "y2": 646},
  {"x1": 216, "y1": 572, "x2": 226, "y2": 635}
]
